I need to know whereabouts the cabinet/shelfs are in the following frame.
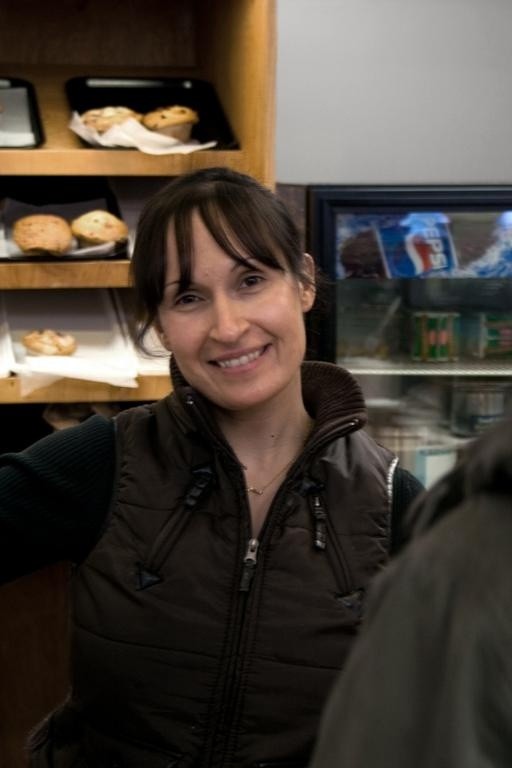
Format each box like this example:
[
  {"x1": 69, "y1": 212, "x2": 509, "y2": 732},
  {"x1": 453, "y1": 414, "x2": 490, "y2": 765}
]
[{"x1": 1, "y1": 0, "x2": 280, "y2": 411}]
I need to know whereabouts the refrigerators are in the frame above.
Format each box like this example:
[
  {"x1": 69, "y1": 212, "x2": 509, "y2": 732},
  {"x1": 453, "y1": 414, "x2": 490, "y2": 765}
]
[{"x1": 277, "y1": 176, "x2": 512, "y2": 500}]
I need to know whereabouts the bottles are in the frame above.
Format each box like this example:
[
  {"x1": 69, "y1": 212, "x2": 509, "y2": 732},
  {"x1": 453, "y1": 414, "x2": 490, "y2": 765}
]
[{"x1": 342, "y1": 211, "x2": 502, "y2": 279}]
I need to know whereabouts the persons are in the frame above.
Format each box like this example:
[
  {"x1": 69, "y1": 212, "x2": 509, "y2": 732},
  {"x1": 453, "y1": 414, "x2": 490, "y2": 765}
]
[
  {"x1": 308, "y1": 416, "x2": 512, "y2": 767},
  {"x1": 0, "y1": 167, "x2": 428, "y2": 768}
]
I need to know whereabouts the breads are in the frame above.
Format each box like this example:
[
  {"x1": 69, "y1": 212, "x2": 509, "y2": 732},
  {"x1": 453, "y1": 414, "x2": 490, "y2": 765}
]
[
  {"x1": 82, "y1": 106, "x2": 141, "y2": 135},
  {"x1": 24, "y1": 331, "x2": 77, "y2": 356},
  {"x1": 141, "y1": 105, "x2": 200, "y2": 142},
  {"x1": 71, "y1": 210, "x2": 130, "y2": 248},
  {"x1": 11, "y1": 213, "x2": 73, "y2": 255}
]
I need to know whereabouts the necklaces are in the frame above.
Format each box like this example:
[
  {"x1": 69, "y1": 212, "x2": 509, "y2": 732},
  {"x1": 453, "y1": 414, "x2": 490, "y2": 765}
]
[{"x1": 238, "y1": 439, "x2": 308, "y2": 494}]
